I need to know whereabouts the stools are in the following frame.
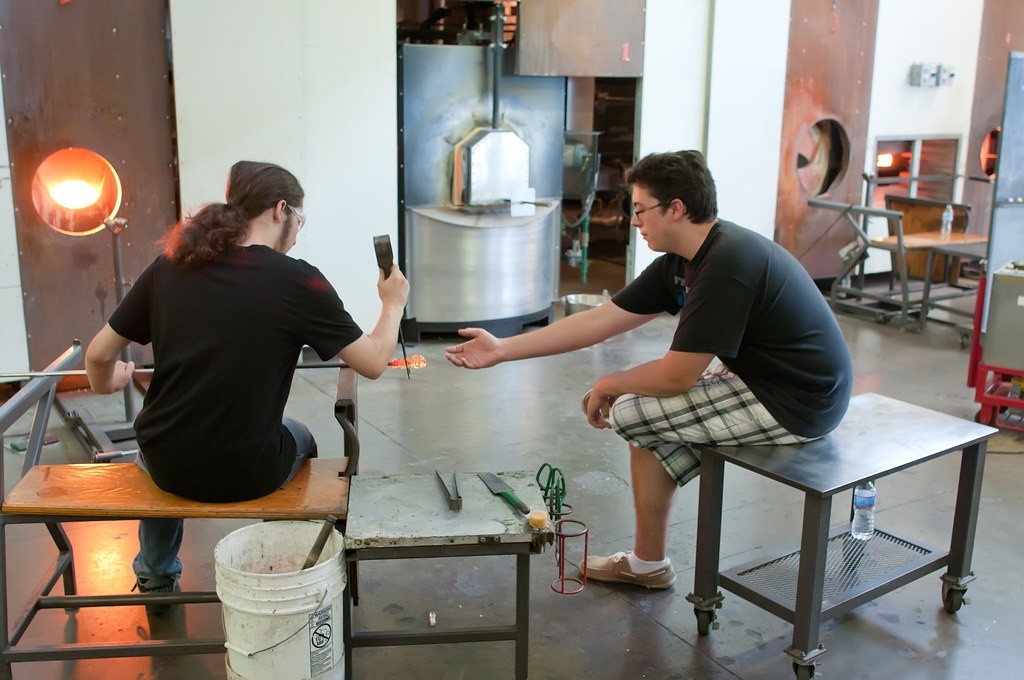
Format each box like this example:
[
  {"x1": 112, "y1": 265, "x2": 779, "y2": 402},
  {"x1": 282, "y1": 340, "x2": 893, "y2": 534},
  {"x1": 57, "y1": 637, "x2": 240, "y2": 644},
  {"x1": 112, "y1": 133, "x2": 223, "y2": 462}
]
[
  {"x1": 344, "y1": 471, "x2": 557, "y2": 679},
  {"x1": 683, "y1": 391, "x2": 1002, "y2": 680},
  {"x1": 0, "y1": 458, "x2": 353, "y2": 680}
]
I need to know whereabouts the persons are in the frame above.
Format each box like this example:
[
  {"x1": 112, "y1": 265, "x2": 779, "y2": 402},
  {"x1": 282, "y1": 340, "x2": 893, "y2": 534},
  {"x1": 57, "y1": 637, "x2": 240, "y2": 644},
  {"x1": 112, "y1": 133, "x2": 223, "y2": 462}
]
[
  {"x1": 446, "y1": 146, "x2": 853, "y2": 593},
  {"x1": 85, "y1": 161, "x2": 409, "y2": 615}
]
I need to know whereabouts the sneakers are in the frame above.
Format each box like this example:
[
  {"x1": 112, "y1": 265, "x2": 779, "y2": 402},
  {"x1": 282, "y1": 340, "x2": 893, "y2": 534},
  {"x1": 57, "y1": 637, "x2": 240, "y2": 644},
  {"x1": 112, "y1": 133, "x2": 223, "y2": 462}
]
[
  {"x1": 135, "y1": 576, "x2": 176, "y2": 611},
  {"x1": 578, "y1": 552, "x2": 677, "y2": 590}
]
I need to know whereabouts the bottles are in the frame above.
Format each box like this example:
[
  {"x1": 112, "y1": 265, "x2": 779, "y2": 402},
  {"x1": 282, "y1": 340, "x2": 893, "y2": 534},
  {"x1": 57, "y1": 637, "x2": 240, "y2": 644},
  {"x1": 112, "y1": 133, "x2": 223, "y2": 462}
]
[
  {"x1": 940, "y1": 204, "x2": 954, "y2": 234},
  {"x1": 850, "y1": 481, "x2": 877, "y2": 540}
]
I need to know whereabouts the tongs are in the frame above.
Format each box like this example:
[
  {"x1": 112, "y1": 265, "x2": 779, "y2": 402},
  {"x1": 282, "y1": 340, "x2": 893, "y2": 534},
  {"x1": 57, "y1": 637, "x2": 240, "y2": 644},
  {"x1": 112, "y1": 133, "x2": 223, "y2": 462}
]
[{"x1": 435, "y1": 470, "x2": 462, "y2": 510}]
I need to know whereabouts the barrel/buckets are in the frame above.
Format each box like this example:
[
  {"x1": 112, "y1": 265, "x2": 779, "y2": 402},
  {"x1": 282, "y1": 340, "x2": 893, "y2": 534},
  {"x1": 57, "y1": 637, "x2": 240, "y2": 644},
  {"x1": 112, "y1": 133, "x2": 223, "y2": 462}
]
[{"x1": 214, "y1": 520, "x2": 348, "y2": 680}]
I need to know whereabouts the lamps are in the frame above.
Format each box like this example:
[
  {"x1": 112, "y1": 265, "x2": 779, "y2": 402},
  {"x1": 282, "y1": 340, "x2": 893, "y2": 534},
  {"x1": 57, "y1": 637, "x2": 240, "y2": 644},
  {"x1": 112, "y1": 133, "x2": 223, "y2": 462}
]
[{"x1": 28, "y1": 149, "x2": 125, "y2": 236}]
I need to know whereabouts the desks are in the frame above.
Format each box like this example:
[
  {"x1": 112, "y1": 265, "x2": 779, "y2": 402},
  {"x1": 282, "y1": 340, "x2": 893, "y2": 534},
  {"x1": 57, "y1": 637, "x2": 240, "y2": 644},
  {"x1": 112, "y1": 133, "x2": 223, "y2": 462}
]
[
  {"x1": 807, "y1": 192, "x2": 987, "y2": 322},
  {"x1": 915, "y1": 241, "x2": 986, "y2": 348}
]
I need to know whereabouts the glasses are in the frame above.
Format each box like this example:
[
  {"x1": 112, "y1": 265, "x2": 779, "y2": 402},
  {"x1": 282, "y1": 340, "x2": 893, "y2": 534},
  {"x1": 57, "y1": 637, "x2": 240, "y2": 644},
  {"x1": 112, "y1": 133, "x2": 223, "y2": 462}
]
[
  {"x1": 287, "y1": 203, "x2": 307, "y2": 231},
  {"x1": 634, "y1": 203, "x2": 661, "y2": 220}
]
